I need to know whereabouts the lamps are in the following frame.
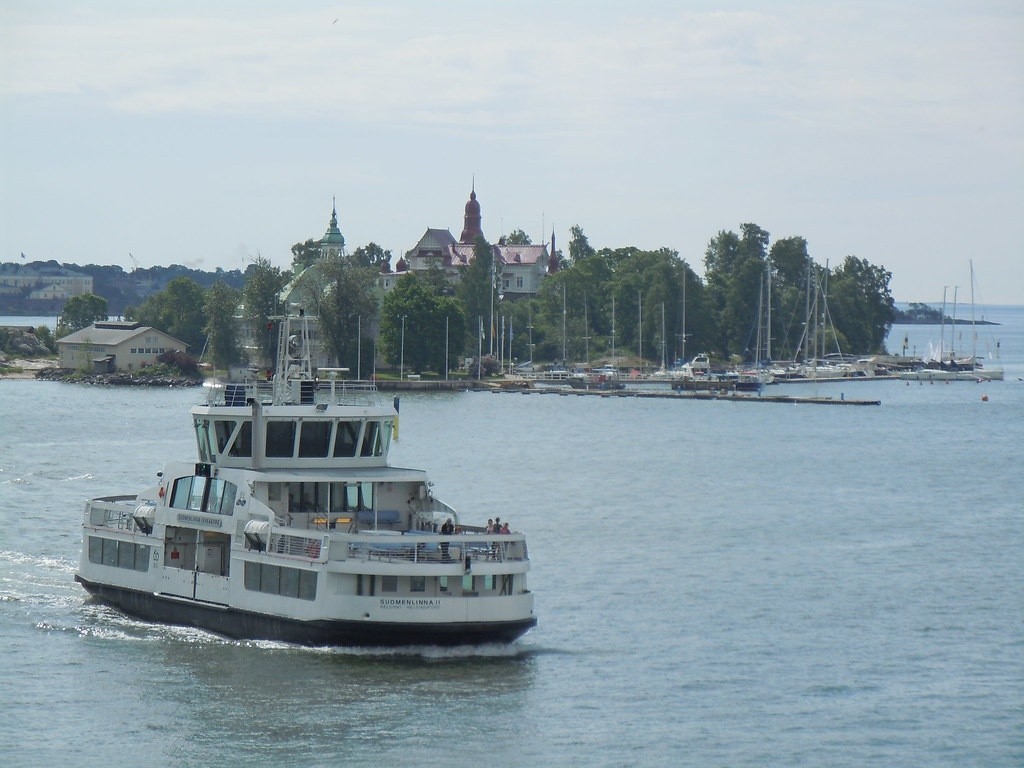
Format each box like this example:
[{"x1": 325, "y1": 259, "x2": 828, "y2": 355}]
[{"x1": 315, "y1": 403, "x2": 328, "y2": 412}]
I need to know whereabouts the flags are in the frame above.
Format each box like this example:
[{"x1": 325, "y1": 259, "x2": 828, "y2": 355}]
[{"x1": 481, "y1": 320, "x2": 513, "y2": 340}]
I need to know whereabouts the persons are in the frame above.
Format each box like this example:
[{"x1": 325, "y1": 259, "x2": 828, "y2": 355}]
[
  {"x1": 440, "y1": 518, "x2": 463, "y2": 560},
  {"x1": 485, "y1": 517, "x2": 511, "y2": 561}
]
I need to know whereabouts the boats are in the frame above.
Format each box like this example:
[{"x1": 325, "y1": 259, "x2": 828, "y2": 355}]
[
  {"x1": 514, "y1": 357, "x2": 1007, "y2": 393},
  {"x1": 74, "y1": 301, "x2": 538, "y2": 648}
]
